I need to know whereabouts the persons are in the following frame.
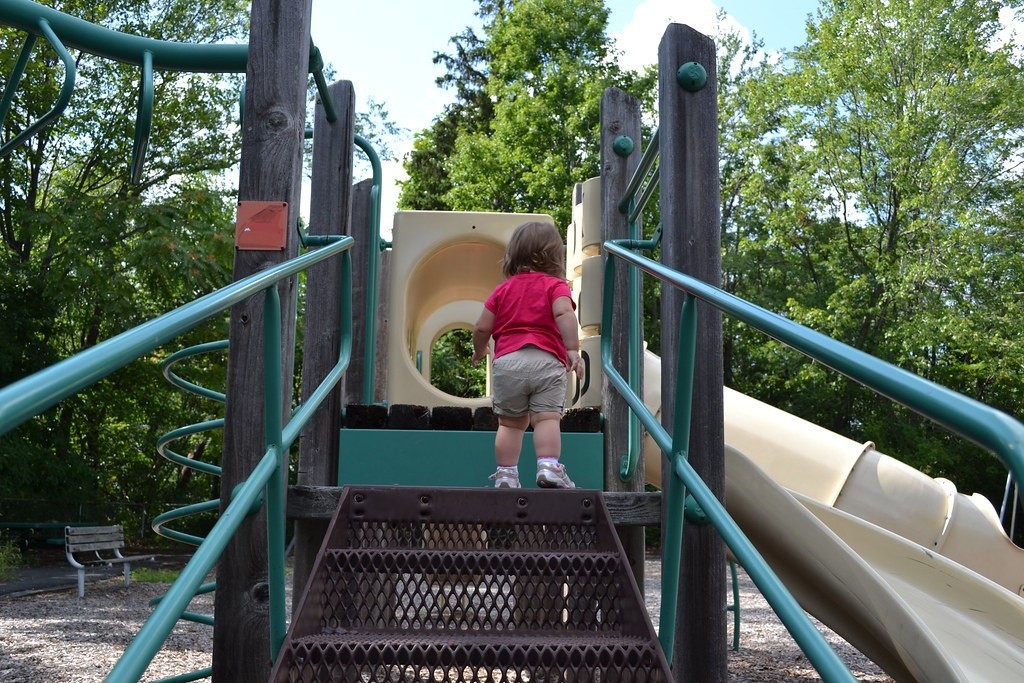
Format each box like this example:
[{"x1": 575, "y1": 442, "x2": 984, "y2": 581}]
[{"x1": 473, "y1": 223, "x2": 584, "y2": 488}]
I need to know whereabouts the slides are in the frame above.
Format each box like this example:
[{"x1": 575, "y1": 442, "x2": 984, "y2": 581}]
[
  {"x1": 641, "y1": 339, "x2": 1024, "y2": 597},
  {"x1": 724, "y1": 445, "x2": 1024, "y2": 683}
]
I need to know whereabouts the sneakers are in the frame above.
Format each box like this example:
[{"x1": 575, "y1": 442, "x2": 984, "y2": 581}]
[
  {"x1": 488, "y1": 469, "x2": 521, "y2": 488},
  {"x1": 536, "y1": 462, "x2": 575, "y2": 489}
]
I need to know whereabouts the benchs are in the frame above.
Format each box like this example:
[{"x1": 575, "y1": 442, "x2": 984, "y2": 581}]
[{"x1": 65, "y1": 526, "x2": 156, "y2": 598}]
